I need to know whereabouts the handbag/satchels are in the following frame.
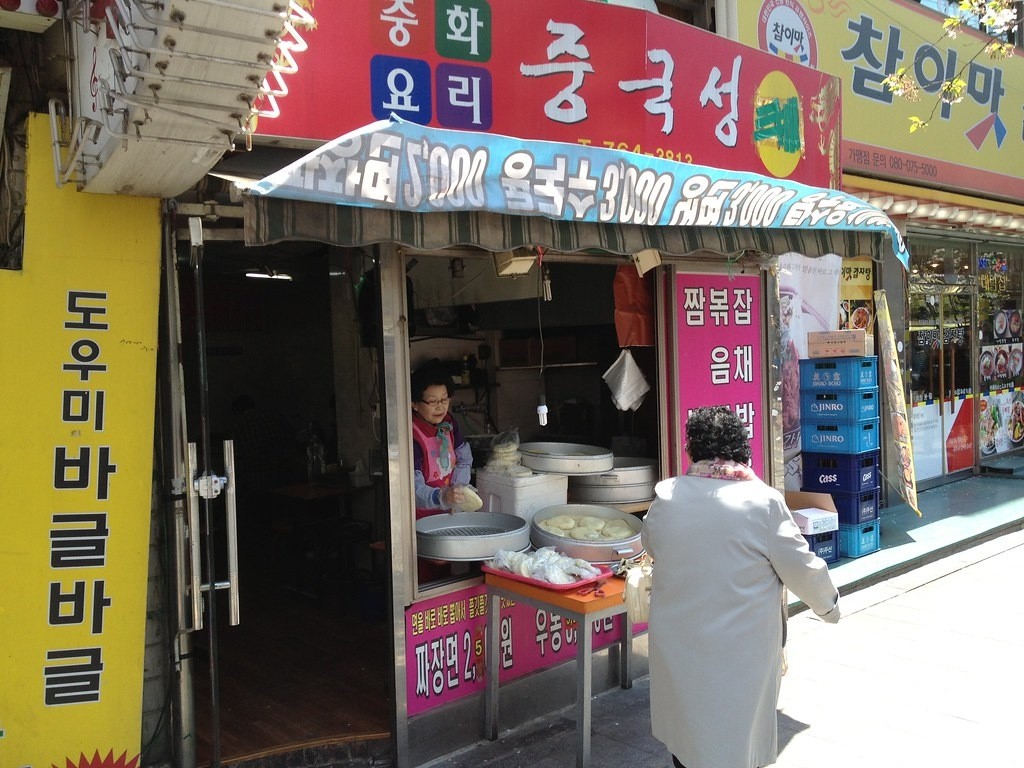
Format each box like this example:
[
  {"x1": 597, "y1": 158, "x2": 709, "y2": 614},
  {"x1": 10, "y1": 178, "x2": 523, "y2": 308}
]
[{"x1": 622, "y1": 554, "x2": 654, "y2": 624}]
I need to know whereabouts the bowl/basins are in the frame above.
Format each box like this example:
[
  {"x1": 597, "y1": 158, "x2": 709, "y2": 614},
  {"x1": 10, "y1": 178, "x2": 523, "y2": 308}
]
[
  {"x1": 995, "y1": 311, "x2": 1022, "y2": 338},
  {"x1": 982, "y1": 440, "x2": 994, "y2": 451},
  {"x1": 979, "y1": 348, "x2": 1023, "y2": 377}
]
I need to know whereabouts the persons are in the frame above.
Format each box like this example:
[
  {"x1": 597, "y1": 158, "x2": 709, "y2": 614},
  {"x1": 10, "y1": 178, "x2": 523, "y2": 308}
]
[
  {"x1": 641, "y1": 406, "x2": 841, "y2": 768},
  {"x1": 411, "y1": 365, "x2": 473, "y2": 585}
]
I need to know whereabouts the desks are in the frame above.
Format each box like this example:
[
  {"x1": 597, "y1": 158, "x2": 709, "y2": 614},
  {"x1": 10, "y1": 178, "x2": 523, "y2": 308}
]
[{"x1": 483, "y1": 573, "x2": 627, "y2": 768}]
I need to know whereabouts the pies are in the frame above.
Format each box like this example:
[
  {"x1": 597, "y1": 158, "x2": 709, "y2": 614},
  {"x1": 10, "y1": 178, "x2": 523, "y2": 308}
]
[
  {"x1": 539, "y1": 515, "x2": 632, "y2": 540},
  {"x1": 489, "y1": 546, "x2": 600, "y2": 584},
  {"x1": 457, "y1": 487, "x2": 483, "y2": 513},
  {"x1": 484, "y1": 441, "x2": 532, "y2": 478}
]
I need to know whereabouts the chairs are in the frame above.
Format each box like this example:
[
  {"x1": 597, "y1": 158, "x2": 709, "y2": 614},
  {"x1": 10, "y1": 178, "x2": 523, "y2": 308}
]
[{"x1": 270, "y1": 484, "x2": 373, "y2": 608}]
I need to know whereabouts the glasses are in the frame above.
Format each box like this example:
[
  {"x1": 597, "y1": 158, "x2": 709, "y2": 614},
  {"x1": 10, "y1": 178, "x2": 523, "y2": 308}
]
[{"x1": 421, "y1": 397, "x2": 452, "y2": 406}]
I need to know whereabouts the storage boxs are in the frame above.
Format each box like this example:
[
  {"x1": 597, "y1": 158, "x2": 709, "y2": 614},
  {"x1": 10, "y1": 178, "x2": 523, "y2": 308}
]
[
  {"x1": 786, "y1": 357, "x2": 881, "y2": 563},
  {"x1": 499, "y1": 336, "x2": 576, "y2": 366},
  {"x1": 476, "y1": 469, "x2": 568, "y2": 523},
  {"x1": 806, "y1": 329, "x2": 875, "y2": 358}
]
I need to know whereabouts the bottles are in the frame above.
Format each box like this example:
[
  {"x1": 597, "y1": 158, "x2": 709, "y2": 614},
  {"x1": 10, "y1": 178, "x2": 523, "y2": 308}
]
[{"x1": 461, "y1": 355, "x2": 471, "y2": 385}]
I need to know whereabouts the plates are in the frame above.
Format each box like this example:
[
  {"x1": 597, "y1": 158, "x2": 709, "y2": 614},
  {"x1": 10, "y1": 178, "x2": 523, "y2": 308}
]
[
  {"x1": 610, "y1": 560, "x2": 639, "y2": 577},
  {"x1": 1006, "y1": 401, "x2": 1024, "y2": 443},
  {"x1": 843, "y1": 301, "x2": 848, "y2": 312},
  {"x1": 852, "y1": 307, "x2": 870, "y2": 329},
  {"x1": 982, "y1": 447, "x2": 995, "y2": 454}
]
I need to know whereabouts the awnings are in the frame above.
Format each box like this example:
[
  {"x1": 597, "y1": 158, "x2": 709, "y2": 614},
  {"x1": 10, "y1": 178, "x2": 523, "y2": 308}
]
[{"x1": 204, "y1": 172, "x2": 882, "y2": 262}]
